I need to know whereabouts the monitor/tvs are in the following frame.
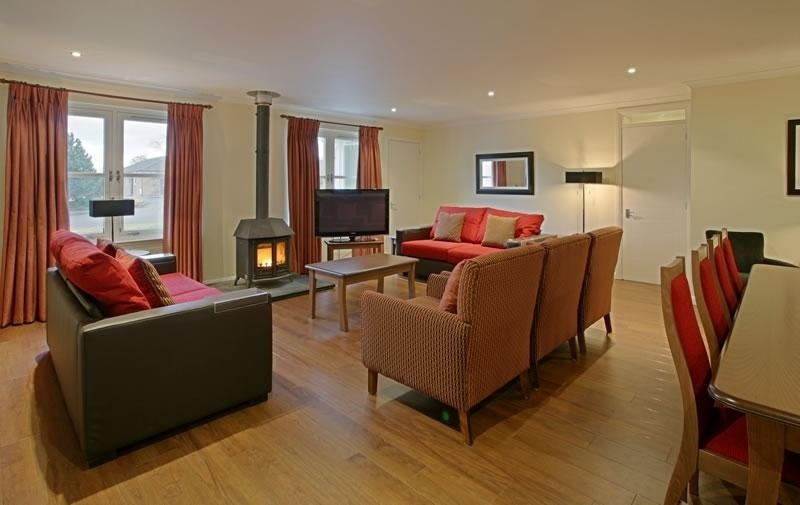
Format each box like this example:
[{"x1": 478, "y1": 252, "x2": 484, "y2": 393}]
[{"x1": 314, "y1": 189, "x2": 389, "y2": 243}]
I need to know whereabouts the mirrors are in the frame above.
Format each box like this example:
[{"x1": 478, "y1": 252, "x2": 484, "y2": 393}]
[{"x1": 476, "y1": 151, "x2": 534, "y2": 194}]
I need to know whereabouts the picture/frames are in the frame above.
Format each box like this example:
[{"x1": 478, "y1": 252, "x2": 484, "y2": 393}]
[{"x1": 785, "y1": 118, "x2": 800, "y2": 197}]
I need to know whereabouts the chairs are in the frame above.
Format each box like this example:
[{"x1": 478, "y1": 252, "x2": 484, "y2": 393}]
[
  {"x1": 439, "y1": 225, "x2": 628, "y2": 390},
  {"x1": 654, "y1": 224, "x2": 800, "y2": 505},
  {"x1": 360, "y1": 244, "x2": 547, "y2": 446}
]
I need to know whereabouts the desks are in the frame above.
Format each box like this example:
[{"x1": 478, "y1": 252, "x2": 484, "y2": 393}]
[
  {"x1": 324, "y1": 238, "x2": 385, "y2": 263},
  {"x1": 125, "y1": 248, "x2": 150, "y2": 256}
]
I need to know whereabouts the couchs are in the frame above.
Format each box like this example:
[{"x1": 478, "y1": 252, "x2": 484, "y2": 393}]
[
  {"x1": 392, "y1": 204, "x2": 545, "y2": 284},
  {"x1": 40, "y1": 252, "x2": 276, "y2": 470}
]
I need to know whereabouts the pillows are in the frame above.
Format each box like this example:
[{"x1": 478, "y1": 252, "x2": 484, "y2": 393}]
[
  {"x1": 110, "y1": 246, "x2": 175, "y2": 309},
  {"x1": 433, "y1": 211, "x2": 467, "y2": 244},
  {"x1": 482, "y1": 211, "x2": 519, "y2": 250},
  {"x1": 92, "y1": 238, "x2": 117, "y2": 257}
]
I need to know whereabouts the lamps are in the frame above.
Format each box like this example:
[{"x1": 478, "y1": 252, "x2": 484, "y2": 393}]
[
  {"x1": 564, "y1": 170, "x2": 603, "y2": 235},
  {"x1": 88, "y1": 197, "x2": 134, "y2": 242}
]
